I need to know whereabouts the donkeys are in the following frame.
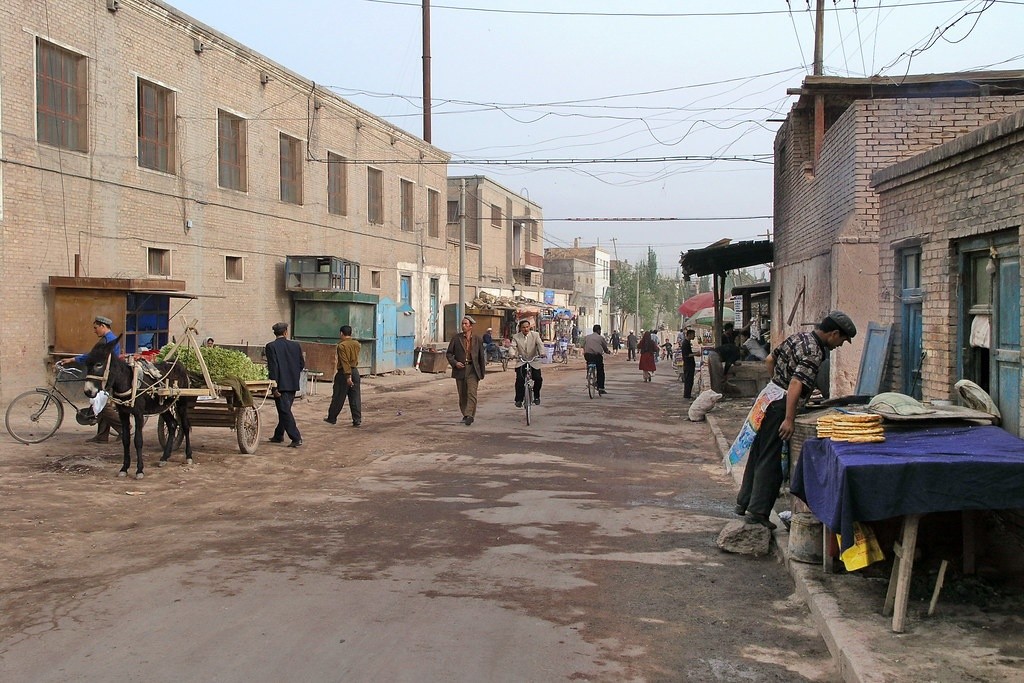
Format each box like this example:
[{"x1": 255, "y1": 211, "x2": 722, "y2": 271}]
[{"x1": 83, "y1": 332, "x2": 198, "y2": 480}]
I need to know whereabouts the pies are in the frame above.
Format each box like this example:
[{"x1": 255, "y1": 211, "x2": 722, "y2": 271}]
[{"x1": 816, "y1": 414, "x2": 885, "y2": 442}]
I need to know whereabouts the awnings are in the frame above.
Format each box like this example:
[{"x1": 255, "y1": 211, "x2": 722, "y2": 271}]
[{"x1": 130, "y1": 290, "x2": 227, "y2": 299}]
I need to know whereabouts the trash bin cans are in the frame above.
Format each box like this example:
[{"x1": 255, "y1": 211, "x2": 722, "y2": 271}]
[{"x1": 414, "y1": 347, "x2": 448, "y2": 374}]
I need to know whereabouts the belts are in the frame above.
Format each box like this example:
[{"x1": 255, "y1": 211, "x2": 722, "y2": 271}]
[{"x1": 465, "y1": 361, "x2": 472, "y2": 365}]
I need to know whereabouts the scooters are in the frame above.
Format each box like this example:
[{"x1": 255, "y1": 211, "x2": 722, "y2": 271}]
[{"x1": 671, "y1": 346, "x2": 684, "y2": 383}]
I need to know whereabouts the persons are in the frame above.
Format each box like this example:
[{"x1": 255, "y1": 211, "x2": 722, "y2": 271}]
[
  {"x1": 446, "y1": 315, "x2": 486, "y2": 425},
  {"x1": 200, "y1": 337, "x2": 214, "y2": 348},
  {"x1": 576, "y1": 321, "x2": 688, "y2": 362},
  {"x1": 637, "y1": 330, "x2": 659, "y2": 383},
  {"x1": 694, "y1": 330, "x2": 712, "y2": 344},
  {"x1": 721, "y1": 319, "x2": 769, "y2": 384},
  {"x1": 735, "y1": 310, "x2": 857, "y2": 529},
  {"x1": 60, "y1": 316, "x2": 133, "y2": 444},
  {"x1": 509, "y1": 319, "x2": 547, "y2": 408},
  {"x1": 265, "y1": 323, "x2": 305, "y2": 447},
  {"x1": 324, "y1": 326, "x2": 362, "y2": 428},
  {"x1": 583, "y1": 325, "x2": 611, "y2": 395},
  {"x1": 681, "y1": 329, "x2": 702, "y2": 399},
  {"x1": 482, "y1": 328, "x2": 493, "y2": 344}
]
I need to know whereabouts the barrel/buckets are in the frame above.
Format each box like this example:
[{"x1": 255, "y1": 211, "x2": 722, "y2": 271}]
[{"x1": 788, "y1": 511, "x2": 839, "y2": 565}]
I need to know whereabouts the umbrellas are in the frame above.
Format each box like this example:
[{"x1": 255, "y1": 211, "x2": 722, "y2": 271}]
[
  {"x1": 678, "y1": 292, "x2": 714, "y2": 318},
  {"x1": 683, "y1": 307, "x2": 735, "y2": 344}
]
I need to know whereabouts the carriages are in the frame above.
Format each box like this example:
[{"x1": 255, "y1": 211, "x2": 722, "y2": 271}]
[{"x1": 83, "y1": 313, "x2": 278, "y2": 481}]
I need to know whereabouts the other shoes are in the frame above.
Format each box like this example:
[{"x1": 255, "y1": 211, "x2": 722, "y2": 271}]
[
  {"x1": 744, "y1": 510, "x2": 778, "y2": 530},
  {"x1": 734, "y1": 504, "x2": 747, "y2": 516},
  {"x1": 462, "y1": 416, "x2": 474, "y2": 425},
  {"x1": 644, "y1": 379, "x2": 647, "y2": 382},
  {"x1": 324, "y1": 417, "x2": 336, "y2": 424},
  {"x1": 85, "y1": 437, "x2": 108, "y2": 444},
  {"x1": 352, "y1": 423, "x2": 361, "y2": 427},
  {"x1": 534, "y1": 398, "x2": 540, "y2": 405},
  {"x1": 116, "y1": 425, "x2": 132, "y2": 441},
  {"x1": 634, "y1": 359, "x2": 637, "y2": 361},
  {"x1": 516, "y1": 401, "x2": 522, "y2": 407},
  {"x1": 649, "y1": 376, "x2": 651, "y2": 381},
  {"x1": 718, "y1": 398, "x2": 732, "y2": 402},
  {"x1": 287, "y1": 439, "x2": 302, "y2": 447},
  {"x1": 501, "y1": 357, "x2": 506, "y2": 359},
  {"x1": 599, "y1": 390, "x2": 608, "y2": 394},
  {"x1": 268, "y1": 438, "x2": 284, "y2": 442},
  {"x1": 627, "y1": 359, "x2": 631, "y2": 361}
]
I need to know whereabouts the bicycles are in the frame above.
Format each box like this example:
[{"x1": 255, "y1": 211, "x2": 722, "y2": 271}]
[
  {"x1": 582, "y1": 350, "x2": 605, "y2": 400},
  {"x1": 552, "y1": 340, "x2": 568, "y2": 364},
  {"x1": 509, "y1": 354, "x2": 548, "y2": 426},
  {"x1": 4, "y1": 358, "x2": 150, "y2": 444}
]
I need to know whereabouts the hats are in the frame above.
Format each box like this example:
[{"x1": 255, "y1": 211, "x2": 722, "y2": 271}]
[
  {"x1": 464, "y1": 316, "x2": 475, "y2": 324},
  {"x1": 829, "y1": 310, "x2": 857, "y2": 344},
  {"x1": 95, "y1": 316, "x2": 113, "y2": 325},
  {"x1": 630, "y1": 330, "x2": 633, "y2": 333},
  {"x1": 272, "y1": 323, "x2": 288, "y2": 331},
  {"x1": 639, "y1": 328, "x2": 644, "y2": 332},
  {"x1": 487, "y1": 328, "x2": 492, "y2": 332}
]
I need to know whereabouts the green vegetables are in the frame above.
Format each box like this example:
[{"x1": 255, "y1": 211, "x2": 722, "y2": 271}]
[{"x1": 156, "y1": 343, "x2": 269, "y2": 382}]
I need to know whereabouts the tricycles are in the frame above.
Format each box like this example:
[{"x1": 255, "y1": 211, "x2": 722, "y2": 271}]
[{"x1": 483, "y1": 343, "x2": 509, "y2": 372}]
